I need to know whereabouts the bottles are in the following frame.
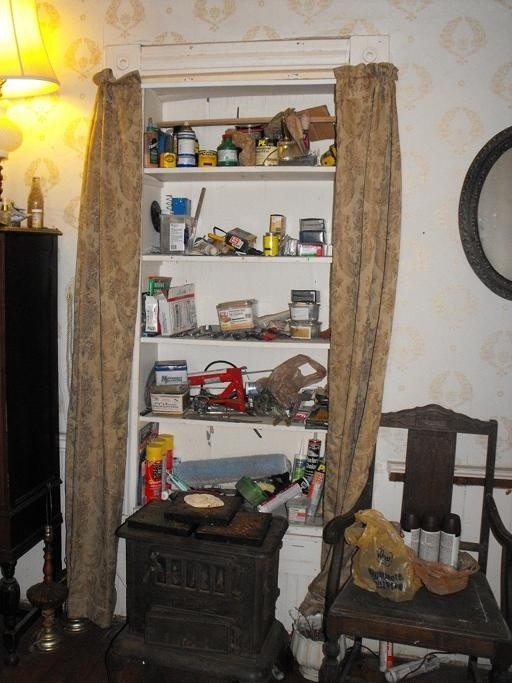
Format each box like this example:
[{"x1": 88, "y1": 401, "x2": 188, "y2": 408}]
[
  {"x1": 144, "y1": 433, "x2": 174, "y2": 504},
  {"x1": 400, "y1": 509, "x2": 462, "y2": 570},
  {"x1": 27, "y1": 177, "x2": 44, "y2": 229},
  {"x1": 177, "y1": 121, "x2": 196, "y2": 167},
  {"x1": 217, "y1": 134, "x2": 239, "y2": 167}
]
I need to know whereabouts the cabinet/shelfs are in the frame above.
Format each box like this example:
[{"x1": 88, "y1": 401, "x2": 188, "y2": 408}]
[{"x1": 128, "y1": 77, "x2": 345, "y2": 536}]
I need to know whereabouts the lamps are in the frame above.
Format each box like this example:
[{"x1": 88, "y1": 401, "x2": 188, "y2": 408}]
[{"x1": 0, "y1": 0, "x2": 63, "y2": 228}]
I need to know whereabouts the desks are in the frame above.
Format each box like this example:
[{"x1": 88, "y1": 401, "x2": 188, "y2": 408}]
[{"x1": 1, "y1": 227, "x2": 66, "y2": 668}]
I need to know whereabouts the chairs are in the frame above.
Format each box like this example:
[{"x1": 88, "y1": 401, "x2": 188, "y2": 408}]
[{"x1": 316, "y1": 403, "x2": 512, "y2": 683}]
